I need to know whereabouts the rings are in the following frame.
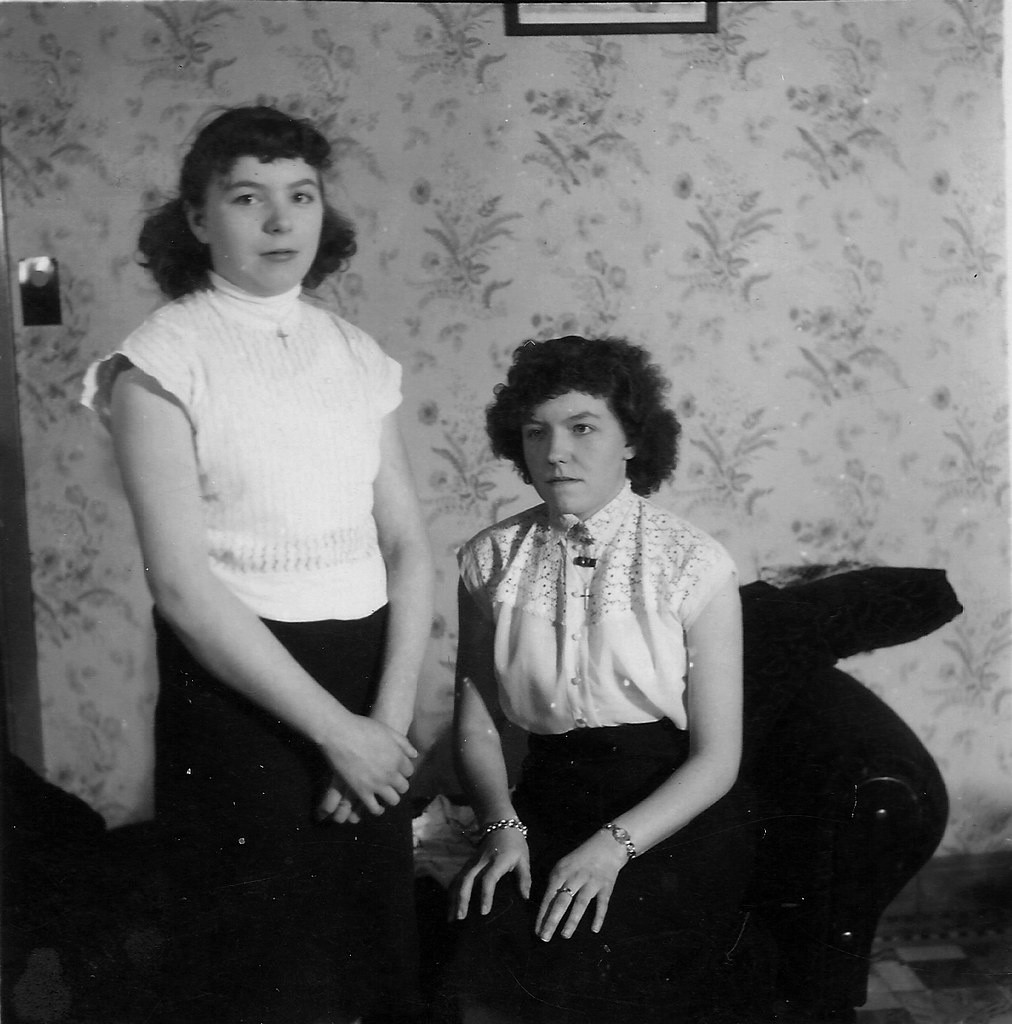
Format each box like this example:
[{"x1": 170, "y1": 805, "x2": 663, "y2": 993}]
[{"x1": 559, "y1": 888, "x2": 574, "y2": 897}]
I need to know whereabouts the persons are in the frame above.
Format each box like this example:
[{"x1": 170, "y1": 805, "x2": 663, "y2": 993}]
[
  {"x1": 79, "y1": 105, "x2": 437, "y2": 1024},
  {"x1": 0, "y1": 748, "x2": 109, "y2": 1024},
  {"x1": 445, "y1": 335, "x2": 744, "y2": 1024}
]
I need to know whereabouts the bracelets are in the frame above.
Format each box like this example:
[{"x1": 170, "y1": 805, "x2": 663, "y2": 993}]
[{"x1": 478, "y1": 818, "x2": 528, "y2": 843}]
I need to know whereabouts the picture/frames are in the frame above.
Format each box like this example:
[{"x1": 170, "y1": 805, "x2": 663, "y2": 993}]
[{"x1": 502, "y1": 3, "x2": 720, "y2": 36}]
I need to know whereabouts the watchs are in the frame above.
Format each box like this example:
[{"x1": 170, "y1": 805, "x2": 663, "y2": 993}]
[{"x1": 603, "y1": 823, "x2": 636, "y2": 860}]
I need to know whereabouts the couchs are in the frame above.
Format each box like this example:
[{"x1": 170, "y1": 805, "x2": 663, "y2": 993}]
[{"x1": 413, "y1": 601, "x2": 950, "y2": 1023}]
